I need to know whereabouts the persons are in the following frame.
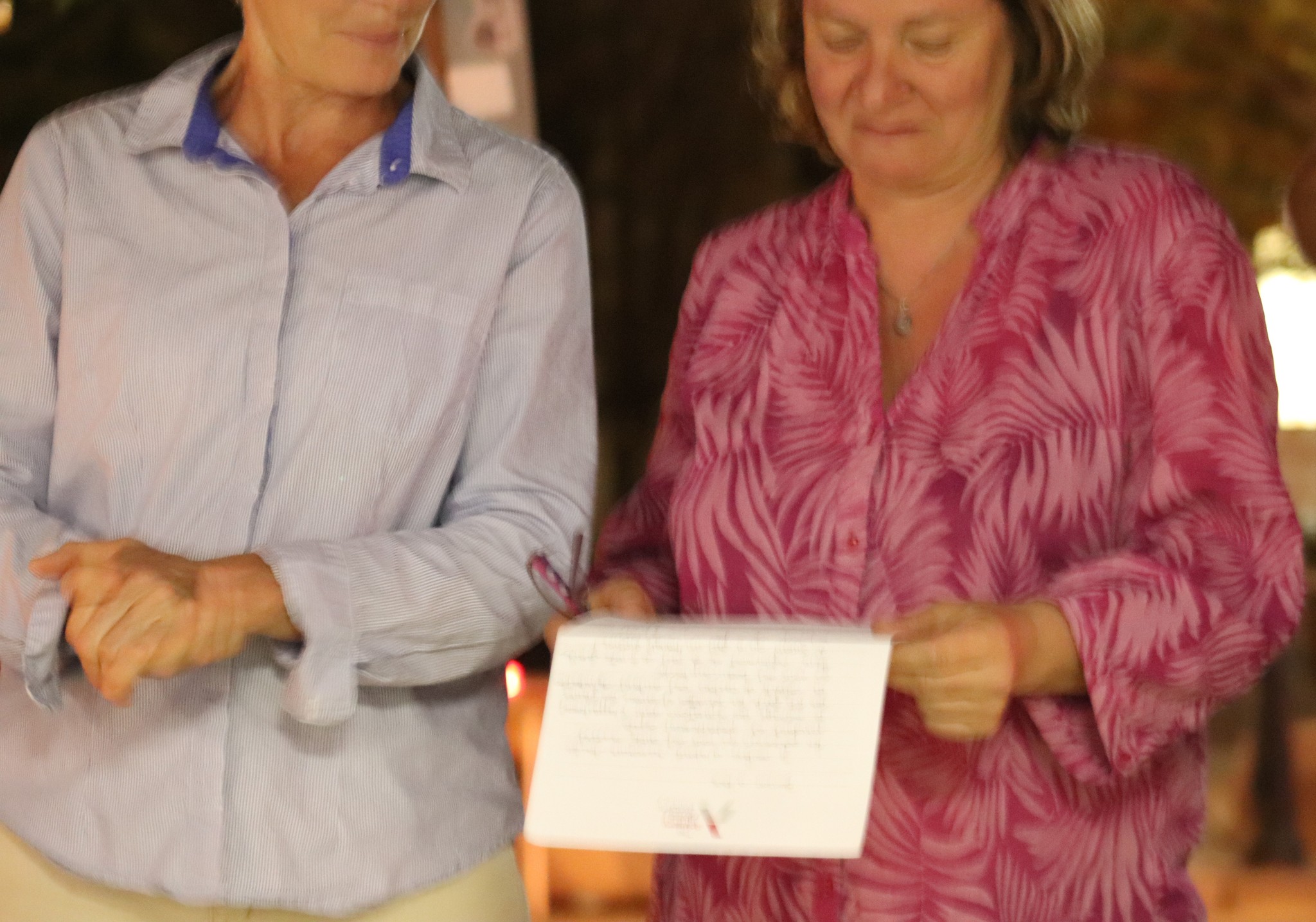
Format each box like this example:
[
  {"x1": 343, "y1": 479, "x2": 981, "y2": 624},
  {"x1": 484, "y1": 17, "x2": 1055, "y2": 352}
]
[
  {"x1": 0, "y1": 0, "x2": 601, "y2": 922},
  {"x1": 531, "y1": 0, "x2": 1311, "y2": 922}
]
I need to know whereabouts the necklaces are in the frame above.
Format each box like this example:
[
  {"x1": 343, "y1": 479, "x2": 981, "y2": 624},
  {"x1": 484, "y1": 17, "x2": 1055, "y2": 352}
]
[{"x1": 874, "y1": 215, "x2": 986, "y2": 336}]
